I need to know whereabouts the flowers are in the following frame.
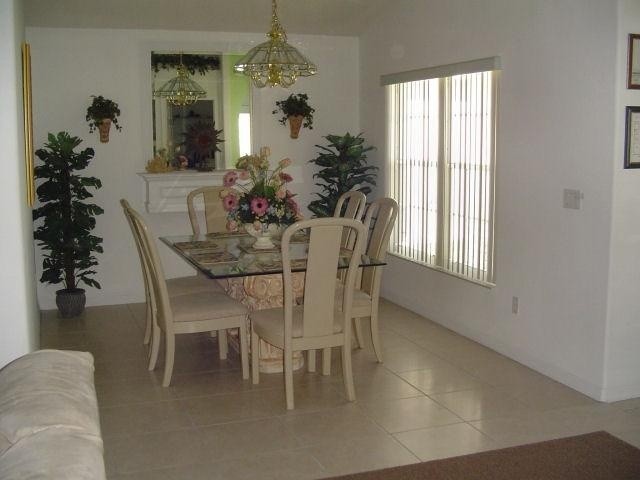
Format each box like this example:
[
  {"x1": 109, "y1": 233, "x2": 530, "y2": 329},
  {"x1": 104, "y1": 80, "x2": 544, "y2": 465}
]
[{"x1": 218, "y1": 145, "x2": 305, "y2": 232}]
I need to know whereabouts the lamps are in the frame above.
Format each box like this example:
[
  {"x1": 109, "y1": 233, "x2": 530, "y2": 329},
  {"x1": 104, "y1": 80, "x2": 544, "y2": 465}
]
[
  {"x1": 232, "y1": 1, "x2": 318, "y2": 90},
  {"x1": 152, "y1": 50, "x2": 207, "y2": 112}
]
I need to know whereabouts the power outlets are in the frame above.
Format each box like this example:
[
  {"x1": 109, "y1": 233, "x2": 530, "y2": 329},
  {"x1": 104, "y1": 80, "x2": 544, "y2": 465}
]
[
  {"x1": 563, "y1": 189, "x2": 583, "y2": 210},
  {"x1": 512, "y1": 296, "x2": 519, "y2": 314}
]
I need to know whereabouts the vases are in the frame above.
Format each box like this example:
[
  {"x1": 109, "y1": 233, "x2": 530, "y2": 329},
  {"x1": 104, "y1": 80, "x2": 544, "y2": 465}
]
[{"x1": 242, "y1": 220, "x2": 280, "y2": 250}]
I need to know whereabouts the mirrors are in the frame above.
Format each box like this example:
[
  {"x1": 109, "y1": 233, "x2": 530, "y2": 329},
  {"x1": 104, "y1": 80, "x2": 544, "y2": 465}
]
[{"x1": 136, "y1": 38, "x2": 261, "y2": 172}]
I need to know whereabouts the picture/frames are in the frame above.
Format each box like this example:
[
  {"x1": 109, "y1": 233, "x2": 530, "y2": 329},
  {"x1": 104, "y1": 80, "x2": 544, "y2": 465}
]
[
  {"x1": 22, "y1": 42, "x2": 34, "y2": 207},
  {"x1": 626, "y1": 33, "x2": 640, "y2": 89},
  {"x1": 623, "y1": 106, "x2": 640, "y2": 168}
]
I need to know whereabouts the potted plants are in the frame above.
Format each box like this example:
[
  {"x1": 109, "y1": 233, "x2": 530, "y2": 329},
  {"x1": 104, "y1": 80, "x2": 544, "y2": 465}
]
[
  {"x1": 86, "y1": 94, "x2": 122, "y2": 144},
  {"x1": 272, "y1": 93, "x2": 315, "y2": 141},
  {"x1": 32, "y1": 130, "x2": 103, "y2": 318}
]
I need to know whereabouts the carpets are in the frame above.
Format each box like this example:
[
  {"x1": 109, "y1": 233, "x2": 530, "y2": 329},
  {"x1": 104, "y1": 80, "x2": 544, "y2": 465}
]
[{"x1": 322, "y1": 430, "x2": 639, "y2": 479}]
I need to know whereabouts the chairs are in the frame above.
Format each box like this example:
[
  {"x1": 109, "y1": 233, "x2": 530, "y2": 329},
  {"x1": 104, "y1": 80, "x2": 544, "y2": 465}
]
[
  {"x1": 306, "y1": 197, "x2": 400, "y2": 373},
  {"x1": 333, "y1": 191, "x2": 366, "y2": 251},
  {"x1": 119, "y1": 197, "x2": 230, "y2": 359},
  {"x1": 186, "y1": 185, "x2": 246, "y2": 236},
  {"x1": 251, "y1": 216, "x2": 369, "y2": 410},
  {"x1": 124, "y1": 205, "x2": 250, "y2": 387}
]
